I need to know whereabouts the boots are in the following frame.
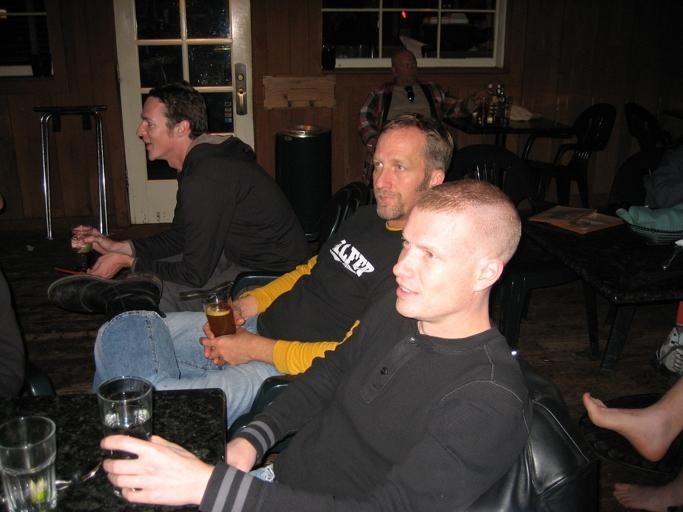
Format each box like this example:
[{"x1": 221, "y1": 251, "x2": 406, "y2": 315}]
[{"x1": 46, "y1": 274, "x2": 168, "y2": 319}]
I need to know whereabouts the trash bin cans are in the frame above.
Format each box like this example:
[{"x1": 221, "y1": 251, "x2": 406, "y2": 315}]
[{"x1": 272, "y1": 126, "x2": 331, "y2": 228}]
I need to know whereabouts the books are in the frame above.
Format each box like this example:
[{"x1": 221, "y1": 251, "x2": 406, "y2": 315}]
[{"x1": 529, "y1": 205, "x2": 624, "y2": 235}]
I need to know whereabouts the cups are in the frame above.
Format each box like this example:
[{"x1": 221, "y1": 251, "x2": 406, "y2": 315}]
[
  {"x1": 97, "y1": 375, "x2": 155, "y2": 497},
  {"x1": 1, "y1": 416, "x2": 58, "y2": 512},
  {"x1": 66, "y1": 221, "x2": 92, "y2": 254},
  {"x1": 202, "y1": 291, "x2": 236, "y2": 337}
]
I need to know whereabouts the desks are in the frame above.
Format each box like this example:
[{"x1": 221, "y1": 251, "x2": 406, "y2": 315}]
[
  {"x1": 2, "y1": 388, "x2": 233, "y2": 509},
  {"x1": 442, "y1": 109, "x2": 563, "y2": 208}
]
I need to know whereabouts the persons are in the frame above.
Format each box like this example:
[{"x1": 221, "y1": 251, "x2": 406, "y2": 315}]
[
  {"x1": 581, "y1": 376, "x2": 683, "y2": 512},
  {"x1": 72, "y1": 80, "x2": 314, "y2": 312},
  {"x1": 46, "y1": 114, "x2": 454, "y2": 429},
  {"x1": 100, "y1": 178, "x2": 533, "y2": 512},
  {"x1": 357, "y1": 49, "x2": 486, "y2": 186}
]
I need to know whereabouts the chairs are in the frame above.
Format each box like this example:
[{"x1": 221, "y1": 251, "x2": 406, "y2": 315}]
[
  {"x1": 226, "y1": 183, "x2": 379, "y2": 306},
  {"x1": 580, "y1": 218, "x2": 682, "y2": 376},
  {"x1": 440, "y1": 144, "x2": 601, "y2": 356},
  {"x1": 521, "y1": 101, "x2": 608, "y2": 208}
]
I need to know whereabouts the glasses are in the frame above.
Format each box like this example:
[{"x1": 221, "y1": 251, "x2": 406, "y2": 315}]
[{"x1": 405, "y1": 85, "x2": 416, "y2": 104}]
